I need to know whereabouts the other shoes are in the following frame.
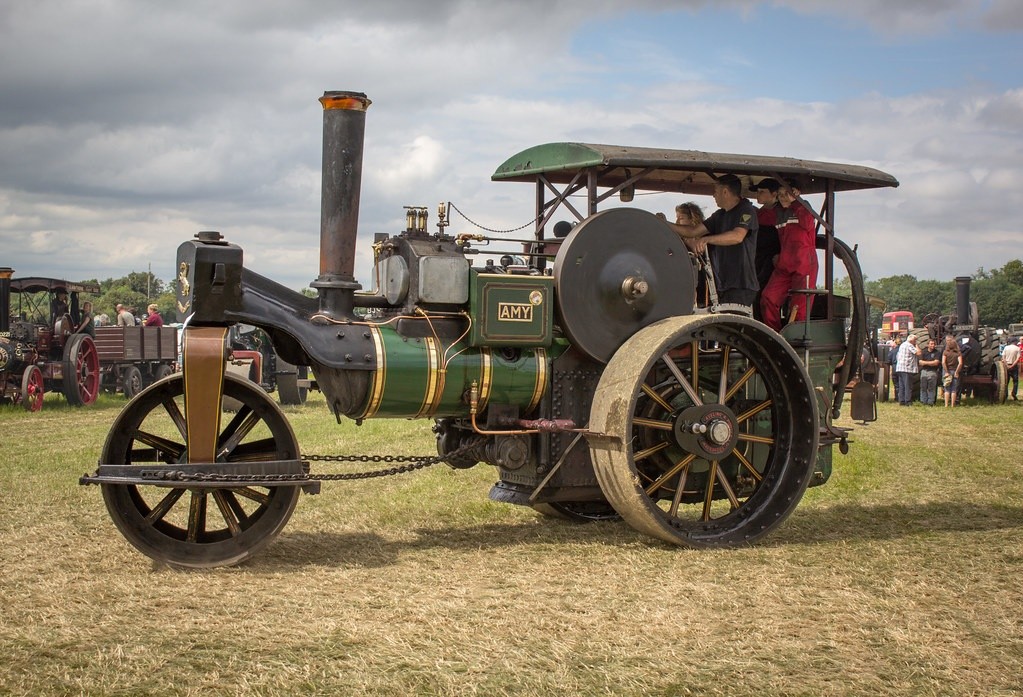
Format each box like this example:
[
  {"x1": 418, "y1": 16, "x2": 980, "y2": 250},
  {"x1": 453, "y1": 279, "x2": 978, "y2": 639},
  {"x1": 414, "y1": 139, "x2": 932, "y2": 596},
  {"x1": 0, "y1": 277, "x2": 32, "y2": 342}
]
[{"x1": 1011, "y1": 392, "x2": 1019, "y2": 401}]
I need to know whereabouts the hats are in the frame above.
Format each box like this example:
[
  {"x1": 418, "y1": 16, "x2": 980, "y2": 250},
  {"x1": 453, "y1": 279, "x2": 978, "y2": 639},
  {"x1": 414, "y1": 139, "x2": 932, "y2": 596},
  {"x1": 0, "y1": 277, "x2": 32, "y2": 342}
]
[
  {"x1": 57, "y1": 288, "x2": 71, "y2": 295},
  {"x1": 748, "y1": 178, "x2": 781, "y2": 191}
]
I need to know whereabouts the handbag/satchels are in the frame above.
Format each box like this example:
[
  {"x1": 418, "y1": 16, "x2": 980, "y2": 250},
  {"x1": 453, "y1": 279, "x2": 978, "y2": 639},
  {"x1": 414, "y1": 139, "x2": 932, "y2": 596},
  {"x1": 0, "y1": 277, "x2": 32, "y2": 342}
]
[{"x1": 942, "y1": 374, "x2": 953, "y2": 386}]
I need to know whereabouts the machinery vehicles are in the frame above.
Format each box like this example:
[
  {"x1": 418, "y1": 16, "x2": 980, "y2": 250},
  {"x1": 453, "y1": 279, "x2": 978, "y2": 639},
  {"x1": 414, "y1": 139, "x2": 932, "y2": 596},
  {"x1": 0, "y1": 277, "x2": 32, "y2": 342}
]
[
  {"x1": 0, "y1": 265, "x2": 181, "y2": 413},
  {"x1": 220, "y1": 326, "x2": 311, "y2": 415}
]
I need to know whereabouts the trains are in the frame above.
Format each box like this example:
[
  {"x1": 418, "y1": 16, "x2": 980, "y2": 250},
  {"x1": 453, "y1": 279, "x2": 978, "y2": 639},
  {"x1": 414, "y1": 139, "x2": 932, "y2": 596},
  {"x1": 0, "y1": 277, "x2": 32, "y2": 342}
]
[
  {"x1": 73, "y1": 87, "x2": 901, "y2": 570},
  {"x1": 905, "y1": 275, "x2": 1008, "y2": 405}
]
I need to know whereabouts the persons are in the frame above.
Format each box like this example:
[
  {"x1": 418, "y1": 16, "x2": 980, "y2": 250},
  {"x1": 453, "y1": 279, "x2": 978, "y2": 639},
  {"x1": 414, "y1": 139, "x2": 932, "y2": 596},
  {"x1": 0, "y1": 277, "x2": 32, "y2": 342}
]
[
  {"x1": 53, "y1": 292, "x2": 69, "y2": 322},
  {"x1": 76, "y1": 302, "x2": 95, "y2": 340},
  {"x1": 1000, "y1": 336, "x2": 1023, "y2": 400},
  {"x1": 256, "y1": 332, "x2": 277, "y2": 392},
  {"x1": 656, "y1": 174, "x2": 819, "y2": 334},
  {"x1": 878, "y1": 334, "x2": 962, "y2": 407},
  {"x1": 116, "y1": 304, "x2": 163, "y2": 327},
  {"x1": 94, "y1": 314, "x2": 112, "y2": 326}
]
